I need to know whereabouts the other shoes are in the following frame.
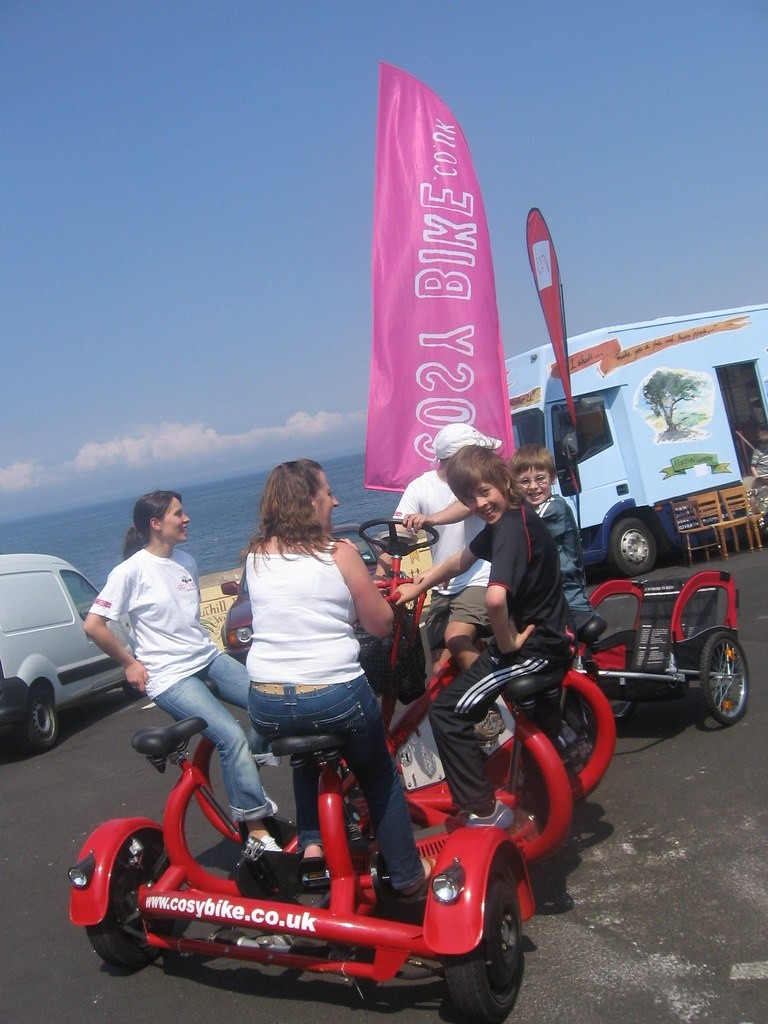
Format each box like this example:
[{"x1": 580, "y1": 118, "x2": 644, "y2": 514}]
[{"x1": 463, "y1": 800, "x2": 516, "y2": 827}]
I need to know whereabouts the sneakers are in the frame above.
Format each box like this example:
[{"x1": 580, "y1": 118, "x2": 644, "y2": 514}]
[
  {"x1": 254, "y1": 834, "x2": 282, "y2": 854},
  {"x1": 261, "y1": 787, "x2": 280, "y2": 817},
  {"x1": 474, "y1": 702, "x2": 506, "y2": 740}
]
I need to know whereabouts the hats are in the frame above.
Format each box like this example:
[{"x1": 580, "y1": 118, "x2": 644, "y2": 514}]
[{"x1": 433, "y1": 424, "x2": 502, "y2": 460}]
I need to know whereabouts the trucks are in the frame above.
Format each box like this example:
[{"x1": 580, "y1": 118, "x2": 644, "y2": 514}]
[{"x1": 503, "y1": 303, "x2": 768, "y2": 580}]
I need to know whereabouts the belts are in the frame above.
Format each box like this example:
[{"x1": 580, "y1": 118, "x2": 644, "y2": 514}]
[{"x1": 250, "y1": 680, "x2": 328, "y2": 695}]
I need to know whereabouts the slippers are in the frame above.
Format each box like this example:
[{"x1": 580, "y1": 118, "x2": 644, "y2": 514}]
[
  {"x1": 300, "y1": 858, "x2": 326, "y2": 873},
  {"x1": 398, "y1": 856, "x2": 438, "y2": 905}
]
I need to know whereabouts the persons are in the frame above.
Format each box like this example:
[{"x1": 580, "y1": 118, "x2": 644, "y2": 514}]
[
  {"x1": 244, "y1": 459, "x2": 437, "y2": 903},
  {"x1": 85, "y1": 491, "x2": 284, "y2": 853},
  {"x1": 393, "y1": 445, "x2": 576, "y2": 830},
  {"x1": 751, "y1": 431, "x2": 768, "y2": 478},
  {"x1": 374, "y1": 422, "x2": 502, "y2": 670},
  {"x1": 750, "y1": 477, "x2": 768, "y2": 529},
  {"x1": 508, "y1": 445, "x2": 598, "y2": 629}
]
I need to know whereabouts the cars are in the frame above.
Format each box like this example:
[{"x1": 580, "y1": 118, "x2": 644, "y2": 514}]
[{"x1": 220, "y1": 523, "x2": 425, "y2": 671}]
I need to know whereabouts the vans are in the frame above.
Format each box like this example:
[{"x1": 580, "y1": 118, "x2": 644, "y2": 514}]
[{"x1": 0, "y1": 553, "x2": 146, "y2": 754}]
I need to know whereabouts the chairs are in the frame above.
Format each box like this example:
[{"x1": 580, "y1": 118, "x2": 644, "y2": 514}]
[
  {"x1": 686, "y1": 490, "x2": 753, "y2": 560},
  {"x1": 669, "y1": 499, "x2": 722, "y2": 567},
  {"x1": 719, "y1": 485, "x2": 768, "y2": 551}
]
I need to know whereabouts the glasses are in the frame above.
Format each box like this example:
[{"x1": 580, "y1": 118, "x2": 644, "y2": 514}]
[{"x1": 520, "y1": 474, "x2": 552, "y2": 486}]
